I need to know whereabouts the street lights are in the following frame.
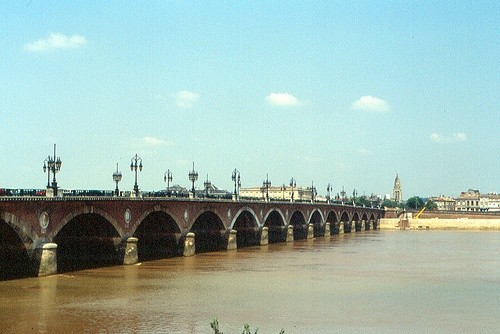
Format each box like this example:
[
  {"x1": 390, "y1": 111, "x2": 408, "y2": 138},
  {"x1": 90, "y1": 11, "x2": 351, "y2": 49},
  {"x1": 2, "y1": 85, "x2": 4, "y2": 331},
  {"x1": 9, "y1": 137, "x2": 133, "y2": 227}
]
[
  {"x1": 260, "y1": 172, "x2": 271, "y2": 202},
  {"x1": 309, "y1": 179, "x2": 315, "y2": 203},
  {"x1": 204, "y1": 173, "x2": 212, "y2": 198},
  {"x1": 112, "y1": 162, "x2": 123, "y2": 196},
  {"x1": 164, "y1": 169, "x2": 174, "y2": 197},
  {"x1": 280, "y1": 183, "x2": 286, "y2": 200},
  {"x1": 377, "y1": 197, "x2": 382, "y2": 209},
  {"x1": 129, "y1": 153, "x2": 143, "y2": 198},
  {"x1": 189, "y1": 160, "x2": 199, "y2": 199},
  {"x1": 341, "y1": 186, "x2": 346, "y2": 205},
  {"x1": 289, "y1": 177, "x2": 298, "y2": 203},
  {"x1": 327, "y1": 183, "x2": 332, "y2": 204},
  {"x1": 231, "y1": 167, "x2": 241, "y2": 201},
  {"x1": 43, "y1": 144, "x2": 62, "y2": 198},
  {"x1": 353, "y1": 188, "x2": 358, "y2": 207},
  {"x1": 369, "y1": 193, "x2": 374, "y2": 208}
]
